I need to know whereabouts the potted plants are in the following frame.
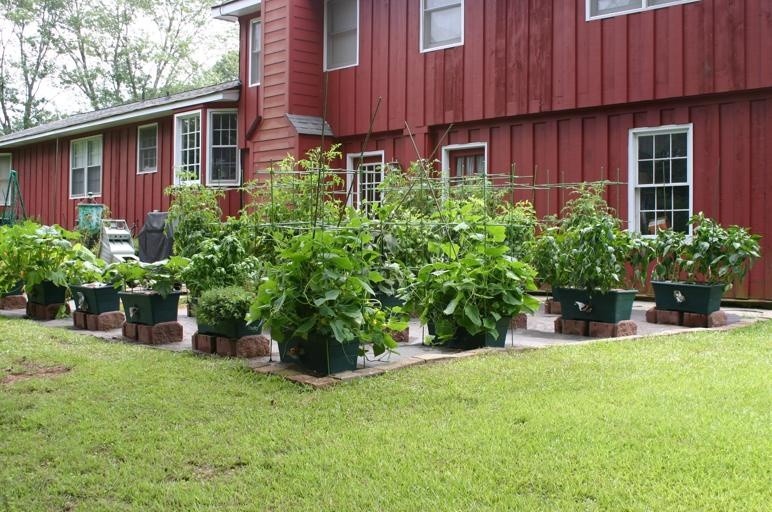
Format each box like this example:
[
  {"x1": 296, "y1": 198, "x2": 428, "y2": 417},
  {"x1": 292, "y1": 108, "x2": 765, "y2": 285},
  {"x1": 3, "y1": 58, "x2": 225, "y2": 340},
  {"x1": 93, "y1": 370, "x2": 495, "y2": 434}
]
[
  {"x1": 270, "y1": 218, "x2": 413, "y2": 380},
  {"x1": 0, "y1": 217, "x2": 42, "y2": 302},
  {"x1": 519, "y1": 179, "x2": 656, "y2": 327},
  {"x1": 522, "y1": 213, "x2": 568, "y2": 302},
  {"x1": 112, "y1": 254, "x2": 192, "y2": 329},
  {"x1": 414, "y1": 215, "x2": 543, "y2": 354},
  {"x1": 638, "y1": 204, "x2": 766, "y2": 316},
  {"x1": 369, "y1": 227, "x2": 410, "y2": 312},
  {"x1": 66, "y1": 247, "x2": 126, "y2": 316},
  {"x1": 19, "y1": 224, "x2": 85, "y2": 308},
  {"x1": 191, "y1": 283, "x2": 268, "y2": 340}
]
